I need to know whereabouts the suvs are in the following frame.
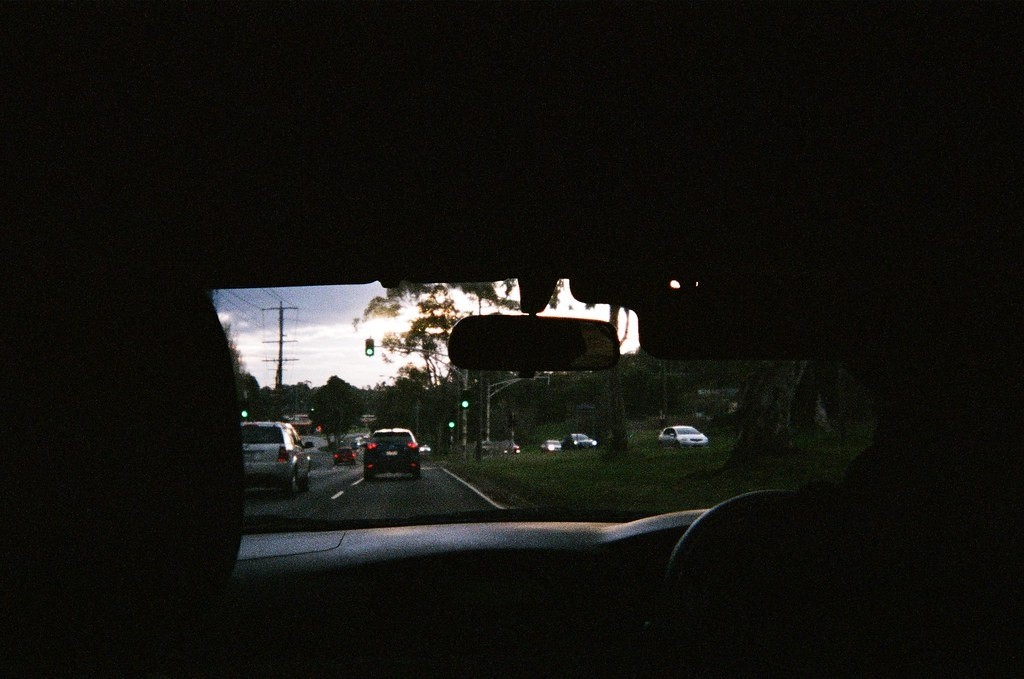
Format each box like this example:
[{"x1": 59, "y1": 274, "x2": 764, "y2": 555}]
[{"x1": 239, "y1": 420, "x2": 315, "y2": 499}]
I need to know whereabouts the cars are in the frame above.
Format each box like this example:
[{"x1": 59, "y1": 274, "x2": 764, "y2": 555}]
[
  {"x1": 542, "y1": 439, "x2": 564, "y2": 451},
  {"x1": 361, "y1": 428, "x2": 422, "y2": 482},
  {"x1": 495, "y1": 440, "x2": 521, "y2": 454},
  {"x1": 331, "y1": 445, "x2": 356, "y2": 466},
  {"x1": 565, "y1": 433, "x2": 598, "y2": 451},
  {"x1": 658, "y1": 425, "x2": 709, "y2": 449}
]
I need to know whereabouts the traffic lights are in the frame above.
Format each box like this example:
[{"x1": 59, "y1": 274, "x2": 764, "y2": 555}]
[
  {"x1": 365, "y1": 338, "x2": 374, "y2": 356},
  {"x1": 447, "y1": 416, "x2": 457, "y2": 433},
  {"x1": 240, "y1": 400, "x2": 253, "y2": 421},
  {"x1": 461, "y1": 388, "x2": 472, "y2": 409}
]
